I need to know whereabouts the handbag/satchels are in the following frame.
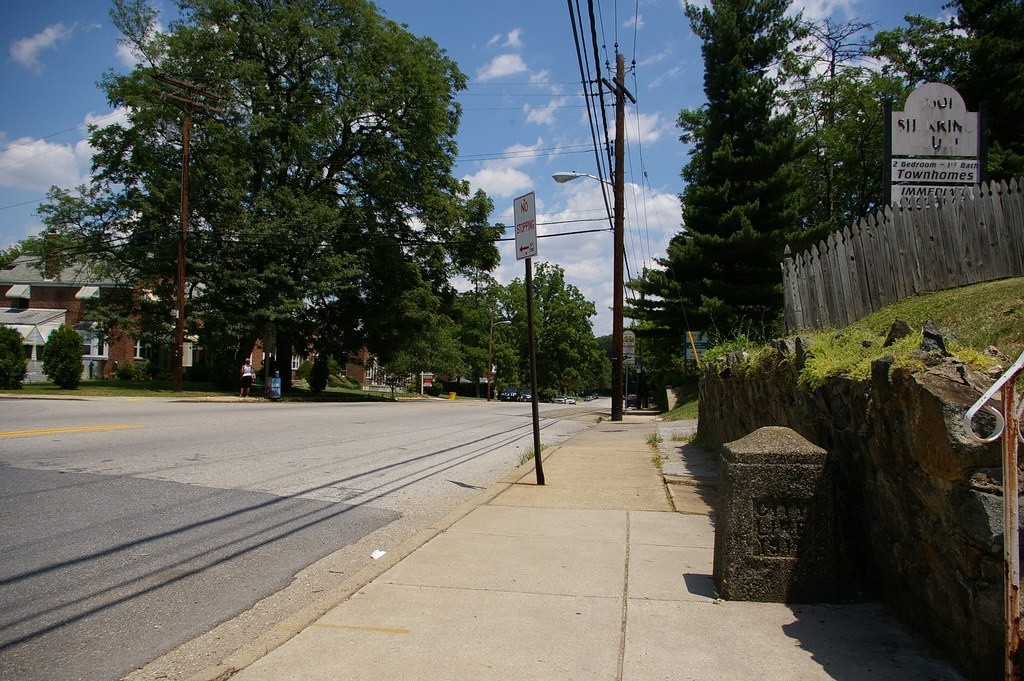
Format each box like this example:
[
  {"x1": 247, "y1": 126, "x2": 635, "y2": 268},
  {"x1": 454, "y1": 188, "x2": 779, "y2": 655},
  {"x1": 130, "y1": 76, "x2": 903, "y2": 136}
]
[{"x1": 252, "y1": 373, "x2": 256, "y2": 379}]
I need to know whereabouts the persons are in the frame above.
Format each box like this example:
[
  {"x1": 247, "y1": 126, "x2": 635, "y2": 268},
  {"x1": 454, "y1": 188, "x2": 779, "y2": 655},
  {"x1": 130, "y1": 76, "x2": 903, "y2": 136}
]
[{"x1": 240, "y1": 358, "x2": 253, "y2": 398}]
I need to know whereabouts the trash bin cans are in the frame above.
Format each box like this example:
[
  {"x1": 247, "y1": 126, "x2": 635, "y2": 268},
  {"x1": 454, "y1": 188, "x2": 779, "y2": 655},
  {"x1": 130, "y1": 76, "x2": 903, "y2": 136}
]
[{"x1": 265, "y1": 376, "x2": 282, "y2": 402}]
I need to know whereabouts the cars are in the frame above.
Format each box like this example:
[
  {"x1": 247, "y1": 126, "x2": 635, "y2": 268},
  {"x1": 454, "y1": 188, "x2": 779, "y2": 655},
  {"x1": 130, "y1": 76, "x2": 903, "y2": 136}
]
[
  {"x1": 582, "y1": 392, "x2": 599, "y2": 401},
  {"x1": 552, "y1": 396, "x2": 567, "y2": 404},
  {"x1": 538, "y1": 394, "x2": 551, "y2": 403},
  {"x1": 519, "y1": 390, "x2": 533, "y2": 401},
  {"x1": 566, "y1": 396, "x2": 577, "y2": 405}
]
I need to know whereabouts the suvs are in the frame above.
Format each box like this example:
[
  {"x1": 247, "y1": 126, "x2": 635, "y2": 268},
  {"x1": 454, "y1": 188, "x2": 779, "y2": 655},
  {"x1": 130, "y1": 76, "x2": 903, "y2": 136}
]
[
  {"x1": 499, "y1": 385, "x2": 521, "y2": 402},
  {"x1": 626, "y1": 393, "x2": 642, "y2": 410}
]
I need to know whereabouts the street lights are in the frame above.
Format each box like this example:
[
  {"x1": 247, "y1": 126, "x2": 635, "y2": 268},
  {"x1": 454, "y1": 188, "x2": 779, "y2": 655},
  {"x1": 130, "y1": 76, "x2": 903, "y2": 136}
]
[
  {"x1": 486, "y1": 312, "x2": 511, "y2": 400},
  {"x1": 553, "y1": 171, "x2": 631, "y2": 422}
]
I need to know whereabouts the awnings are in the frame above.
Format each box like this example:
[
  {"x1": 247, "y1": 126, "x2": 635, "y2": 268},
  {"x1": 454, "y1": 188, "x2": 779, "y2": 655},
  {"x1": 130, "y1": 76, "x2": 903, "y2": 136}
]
[
  {"x1": 5, "y1": 285, "x2": 31, "y2": 299},
  {"x1": 75, "y1": 287, "x2": 100, "y2": 299}
]
